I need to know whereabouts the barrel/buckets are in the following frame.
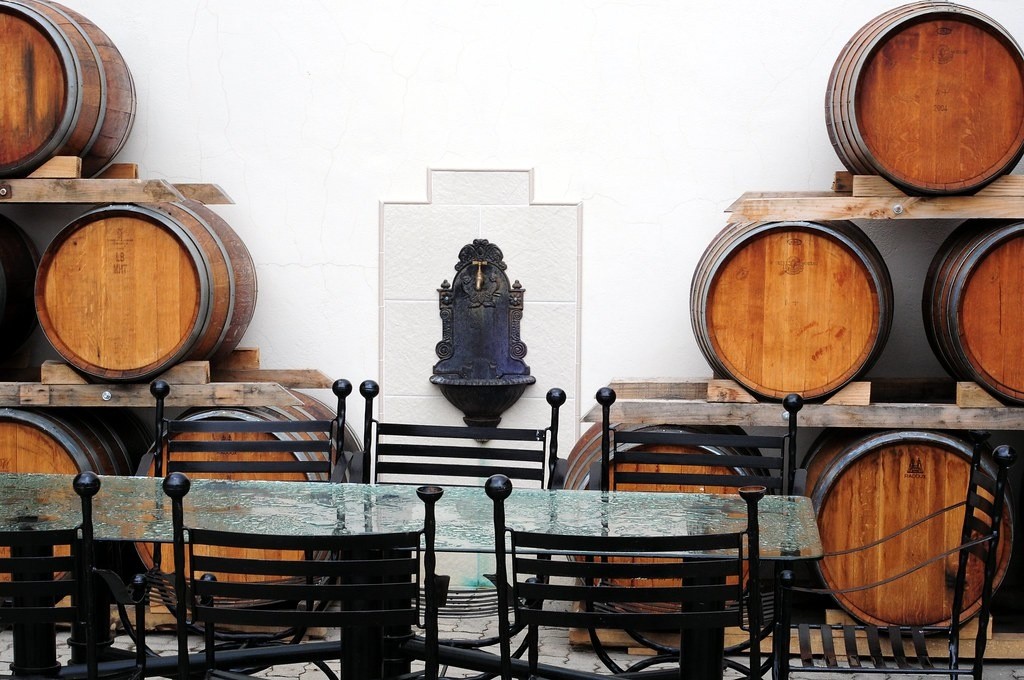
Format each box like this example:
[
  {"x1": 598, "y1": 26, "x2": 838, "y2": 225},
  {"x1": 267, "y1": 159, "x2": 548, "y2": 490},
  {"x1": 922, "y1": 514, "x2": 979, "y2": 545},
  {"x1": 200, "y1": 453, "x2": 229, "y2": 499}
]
[
  {"x1": 0, "y1": 406, "x2": 155, "y2": 606},
  {"x1": 921, "y1": 218, "x2": 1024, "y2": 403},
  {"x1": 801, "y1": 428, "x2": 1014, "y2": 636},
  {"x1": 689, "y1": 222, "x2": 893, "y2": 403},
  {"x1": 825, "y1": 0, "x2": 1024, "y2": 198},
  {"x1": 133, "y1": 389, "x2": 364, "y2": 634},
  {"x1": 34, "y1": 198, "x2": 258, "y2": 382},
  {"x1": 563, "y1": 422, "x2": 775, "y2": 613},
  {"x1": 0, "y1": 0, "x2": 137, "y2": 179}
]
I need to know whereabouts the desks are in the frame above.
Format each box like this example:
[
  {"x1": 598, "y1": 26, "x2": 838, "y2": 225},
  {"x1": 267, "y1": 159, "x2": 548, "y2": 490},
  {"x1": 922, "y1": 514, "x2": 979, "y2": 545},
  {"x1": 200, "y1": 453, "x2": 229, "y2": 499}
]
[{"x1": 1, "y1": 473, "x2": 824, "y2": 680}]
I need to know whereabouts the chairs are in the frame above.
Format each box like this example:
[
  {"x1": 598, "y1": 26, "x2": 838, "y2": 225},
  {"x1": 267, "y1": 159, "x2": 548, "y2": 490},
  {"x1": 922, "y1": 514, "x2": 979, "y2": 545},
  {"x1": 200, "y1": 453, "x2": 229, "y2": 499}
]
[{"x1": 0, "y1": 380, "x2": 1018, "y2": 680}]
